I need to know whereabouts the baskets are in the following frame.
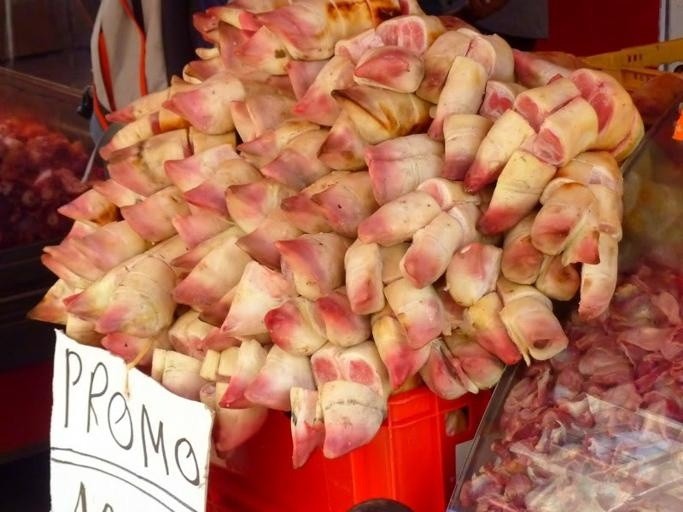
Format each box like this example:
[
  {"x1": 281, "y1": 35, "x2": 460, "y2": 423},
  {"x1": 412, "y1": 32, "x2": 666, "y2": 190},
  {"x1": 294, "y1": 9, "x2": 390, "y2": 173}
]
[{"x1": 581, "y1": 39, "x2": 681, "y2": 96}]
